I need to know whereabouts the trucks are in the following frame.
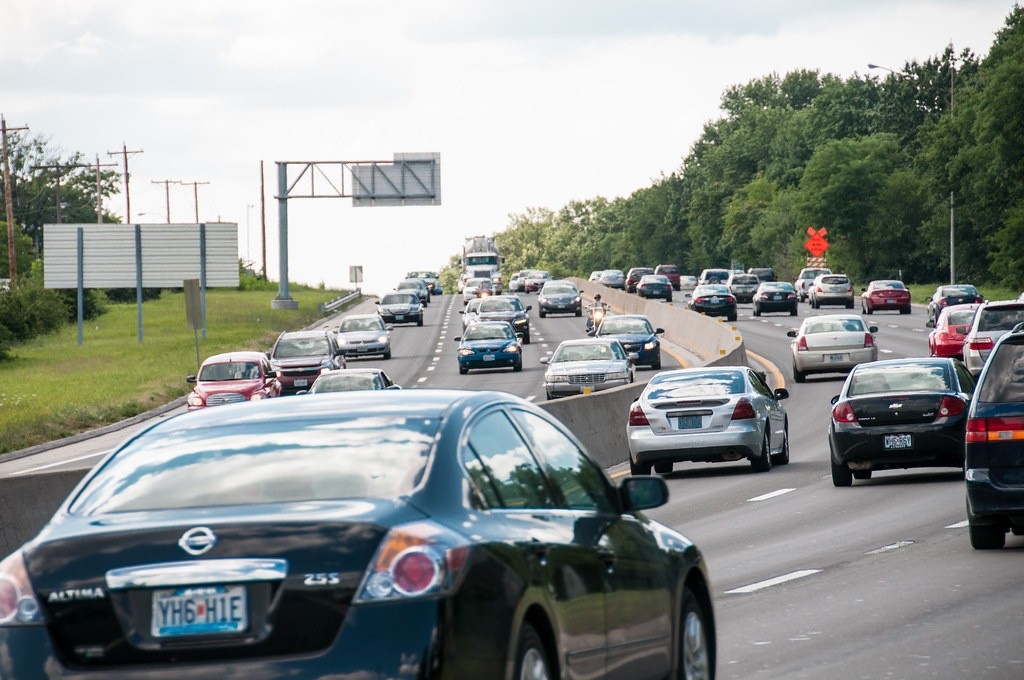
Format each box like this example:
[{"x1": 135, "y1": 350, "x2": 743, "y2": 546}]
[{"x1": 462, "y1": 236, "x2": 505, "y2": 295}]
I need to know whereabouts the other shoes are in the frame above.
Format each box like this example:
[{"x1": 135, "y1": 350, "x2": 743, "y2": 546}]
[{"x1": 586, "y1": 330, "x2": 590, "y2": 332}]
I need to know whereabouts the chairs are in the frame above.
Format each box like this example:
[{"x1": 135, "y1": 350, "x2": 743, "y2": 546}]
[{"x1": 985, "y1": 312, "x2": 1000, "y2": 328}]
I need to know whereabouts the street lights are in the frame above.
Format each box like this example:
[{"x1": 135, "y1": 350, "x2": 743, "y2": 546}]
[{"x1": 868, "y1": 65, "x2": 955, "y2": 285}]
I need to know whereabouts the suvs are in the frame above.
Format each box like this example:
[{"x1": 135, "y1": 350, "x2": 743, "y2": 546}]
[
  {"x1": 965, "y1": 322, "x2": 1024, "y2": 549},
  {"x1": 956, "y1": 300, "x2": 1024, "y2": 379},
  {"x1": 697, "y1": 267, "x2": 777, "y2": 303}
]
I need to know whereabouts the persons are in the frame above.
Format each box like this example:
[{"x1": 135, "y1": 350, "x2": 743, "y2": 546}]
[
  {"x1": 238, "y1": 362, "x2": 256, "y2": 379},
  {"x1": 586, "y1": 294, "x2": 611, "y2": 331}
]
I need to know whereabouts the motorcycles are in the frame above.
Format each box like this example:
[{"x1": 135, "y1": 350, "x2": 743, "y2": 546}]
[{"x1": 586, "y1": 307, "x2": 609, "y2": 332}]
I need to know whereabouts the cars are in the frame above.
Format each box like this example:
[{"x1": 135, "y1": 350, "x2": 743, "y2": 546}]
[
  {"x1": 787, "y1": 314, "x2": 879, "y2": 384},
  {"x1": 0, "y1": 389, "x2": 716, "y2": 680},
  {"x1": 626, "y1": 366, "x2": 789, "y2": 477},
  {"x1": 267, "y1": 271, "x2": 444, "y2": 396},
  {"x1": 540, "y1": 339, "x2": 639, "y2": 400},
  {"x1": 861, "y1": 280, "x2": 912, "y2": 315},
  {"x1": 588, "y1": 315, "x2": 665, "y2": 369},
  {"x1": 751, "y1": 282, "x2": 797, "y2": 316},
  {"x1": 793, "y1": 267, "x2": 833, "y2": 302},
  {"x1": 807, "y1": 274, "x2": 855, "y2": 309},
  {"x1": 634, "y1": 275, "x2": 673, "y2": 302},
  {"x1": 453, "y1": 269, "x2": 584, "y2": 373},
  {"x1": 828, "y1": 357, "x2": 978, "y2": 487},
  {"x1": 685, "y1": 284, "x2": 738, "y2": 321},
  {"x1": 186, "y1": 351, "x2": 282, "y2": 412},
  {"x1": 589, "y1": 264, "x2": 696, "y2": 293},
  {"x1": 925, "y1": 285, "x2": 983, "y2": 357}
]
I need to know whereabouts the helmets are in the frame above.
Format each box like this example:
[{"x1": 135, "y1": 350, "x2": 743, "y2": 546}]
[{"x1": 594, "y1": 294, "x2": 601, "y2": 299}]
